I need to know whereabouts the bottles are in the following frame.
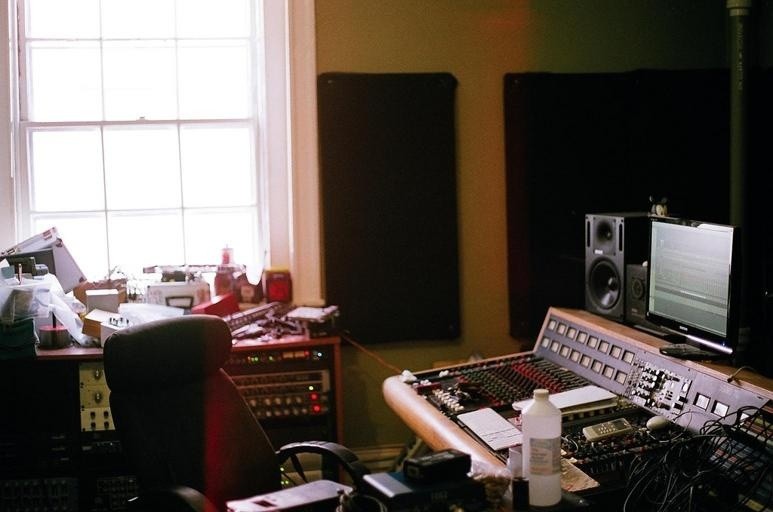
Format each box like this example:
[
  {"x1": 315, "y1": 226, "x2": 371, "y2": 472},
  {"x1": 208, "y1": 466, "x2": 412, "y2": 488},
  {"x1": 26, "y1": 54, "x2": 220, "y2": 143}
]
[{"x1": 521, "y1": 387, "x2": 562, "y2": 507}]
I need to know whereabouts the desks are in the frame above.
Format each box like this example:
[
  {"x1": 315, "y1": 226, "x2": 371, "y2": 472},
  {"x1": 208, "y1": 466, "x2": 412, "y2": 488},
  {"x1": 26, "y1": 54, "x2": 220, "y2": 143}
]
[{"x1": 33, "y1": 332, "x2": 348, "y2": 485}]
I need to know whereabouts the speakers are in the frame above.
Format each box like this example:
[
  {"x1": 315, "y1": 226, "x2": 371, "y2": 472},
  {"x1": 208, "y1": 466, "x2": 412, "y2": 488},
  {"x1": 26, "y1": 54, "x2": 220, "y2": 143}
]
[
  {"x1": 626, "y1": 264, "x2": 652, "y2": 325},
  {"x1": 586, "y1": 213, "x2": 650, "y2": 322}
]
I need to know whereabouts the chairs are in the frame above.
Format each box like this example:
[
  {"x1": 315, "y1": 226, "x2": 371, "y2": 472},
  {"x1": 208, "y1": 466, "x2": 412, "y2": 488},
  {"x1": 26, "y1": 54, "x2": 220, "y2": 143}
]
[{"x1": 103, "y1": 314, "x2": 379, "y2": 512}]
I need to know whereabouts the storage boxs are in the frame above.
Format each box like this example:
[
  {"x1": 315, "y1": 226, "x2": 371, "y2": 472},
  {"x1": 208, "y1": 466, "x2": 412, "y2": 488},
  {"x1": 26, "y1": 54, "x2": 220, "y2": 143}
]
[
  {"x1": 85, "y1": 288, "x2": 118, "y2": 314},
  {"x1": 146, "y1": 281, "x2": 210, "y2": 307}
]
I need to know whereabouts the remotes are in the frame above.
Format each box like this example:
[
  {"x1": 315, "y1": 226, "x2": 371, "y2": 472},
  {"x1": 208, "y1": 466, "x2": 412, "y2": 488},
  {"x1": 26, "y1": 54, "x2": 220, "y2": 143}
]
[{"x1": 582, "y1": 418, "x2": 632, "y2": 441}]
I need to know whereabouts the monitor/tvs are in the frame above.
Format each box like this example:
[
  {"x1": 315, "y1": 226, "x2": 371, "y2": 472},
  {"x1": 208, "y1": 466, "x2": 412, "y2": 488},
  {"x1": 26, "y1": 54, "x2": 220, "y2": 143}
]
[{"x1": 645, "y1": 218, "x2": 737, "y2": 360}]
